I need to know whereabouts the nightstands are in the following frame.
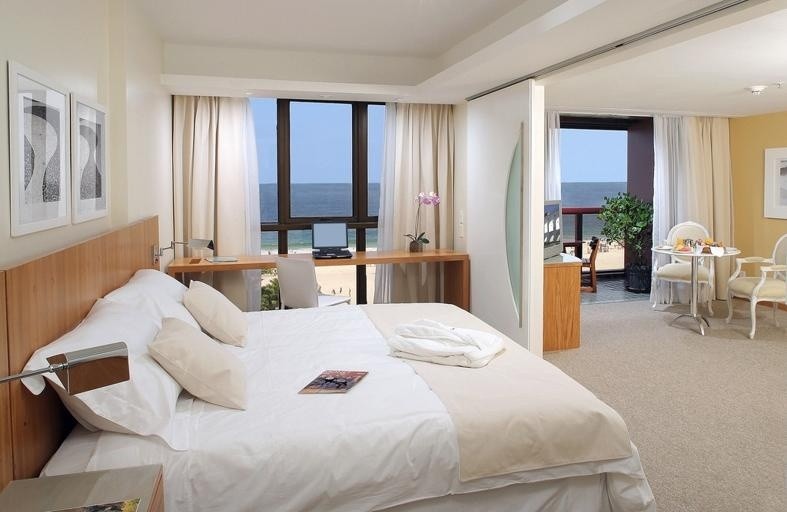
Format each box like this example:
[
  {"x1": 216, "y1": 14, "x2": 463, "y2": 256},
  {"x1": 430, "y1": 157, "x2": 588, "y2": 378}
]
[{"x1": 1, "y1": 464, "x2": 164, "y2": 511}]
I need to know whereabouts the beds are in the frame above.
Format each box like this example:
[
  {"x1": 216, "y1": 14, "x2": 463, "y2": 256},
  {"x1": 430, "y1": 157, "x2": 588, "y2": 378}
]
[{"x1": 1, "y1": 214, "x2": 654, "y2": 512}]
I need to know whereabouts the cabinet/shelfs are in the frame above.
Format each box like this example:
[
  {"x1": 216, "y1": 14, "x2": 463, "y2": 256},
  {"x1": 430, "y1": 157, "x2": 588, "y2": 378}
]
[{"x1": 543, "y1": 252, "x2": 583, "y2": 351}]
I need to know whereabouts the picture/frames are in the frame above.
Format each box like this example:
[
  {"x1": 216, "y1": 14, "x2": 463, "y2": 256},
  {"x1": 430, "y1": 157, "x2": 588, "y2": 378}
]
[
  {"x1": 8, "y1": 60, "x2": 71, "y2": 238},
  {"x1": 763, "y1": 147, "x2": 787, "y2": 220},
  {"x1": 70, "y1": 92, "x2": 111, "y2": 224}
]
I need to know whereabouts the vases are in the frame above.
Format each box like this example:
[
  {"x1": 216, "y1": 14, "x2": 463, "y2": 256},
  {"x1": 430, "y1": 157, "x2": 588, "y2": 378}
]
[{"x1": 410, "y1": 241, "x2": 423, "y2": 252}]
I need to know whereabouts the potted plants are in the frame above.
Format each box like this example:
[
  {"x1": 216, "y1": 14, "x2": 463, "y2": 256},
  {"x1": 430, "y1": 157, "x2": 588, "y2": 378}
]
[{"x1": 597, "y1": 192, "x2": 654, "y2": 293}]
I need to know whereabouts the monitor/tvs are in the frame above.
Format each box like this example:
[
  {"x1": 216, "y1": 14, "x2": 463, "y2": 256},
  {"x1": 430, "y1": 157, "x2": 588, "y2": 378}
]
[{"x1": 544, "y1": 199, "x2": 563, "y2": 262}]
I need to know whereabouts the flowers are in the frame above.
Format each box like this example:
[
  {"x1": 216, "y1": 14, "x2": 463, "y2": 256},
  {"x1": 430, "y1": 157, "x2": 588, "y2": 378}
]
[{"x1": 403, "y1": 190, "x2": 441, "y2": 243}]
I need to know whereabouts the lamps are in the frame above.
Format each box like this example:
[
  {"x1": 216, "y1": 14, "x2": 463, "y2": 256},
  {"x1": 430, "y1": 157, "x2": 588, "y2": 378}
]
[
  {"x1": 153, "y1": 239, "x2": 215, "y2": 265},
  {"x1": 1, "y1": 341, "x2": 129, "y2": 395}
]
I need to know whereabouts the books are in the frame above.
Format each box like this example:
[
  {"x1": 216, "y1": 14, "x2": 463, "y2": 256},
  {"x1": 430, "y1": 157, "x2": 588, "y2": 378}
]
[{"x1": 297, "y1": 369, "x2": 369, "y2": 395}]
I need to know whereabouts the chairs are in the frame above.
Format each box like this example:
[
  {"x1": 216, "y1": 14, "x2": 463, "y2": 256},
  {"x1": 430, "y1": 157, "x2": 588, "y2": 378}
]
[
  {"x1": 650, "y1": 221, "x2": 715, "y2": 317},
  {"x1": 726, "y1": 233, "x2": 787, "y2": 340},
  {"x1": 276, "y1": 257, "x2": 352, "y2": 310},
  {"x1": 581, "y1": 236, "x2": 599, "y2": 293}
]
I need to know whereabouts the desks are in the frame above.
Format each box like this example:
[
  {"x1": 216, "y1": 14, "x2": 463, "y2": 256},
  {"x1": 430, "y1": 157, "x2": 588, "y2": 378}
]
[
  {"x1": 167, "y1": 249, "x2": 470, "y2": 311},
  {"x1": 650, "y1": 246, "x2": 741, "y2": 336}
]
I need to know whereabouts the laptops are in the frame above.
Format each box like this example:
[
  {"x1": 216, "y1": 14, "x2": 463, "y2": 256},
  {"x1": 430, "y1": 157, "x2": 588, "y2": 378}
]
[{"x1": 311, "y1": 221, "x2": 352, "y2": 258}]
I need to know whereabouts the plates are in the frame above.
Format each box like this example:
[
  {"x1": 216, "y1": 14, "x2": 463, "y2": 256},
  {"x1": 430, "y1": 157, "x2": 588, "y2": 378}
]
[{"x1": 657, "y1": 243, "x2": 737, "y2": 253}]
